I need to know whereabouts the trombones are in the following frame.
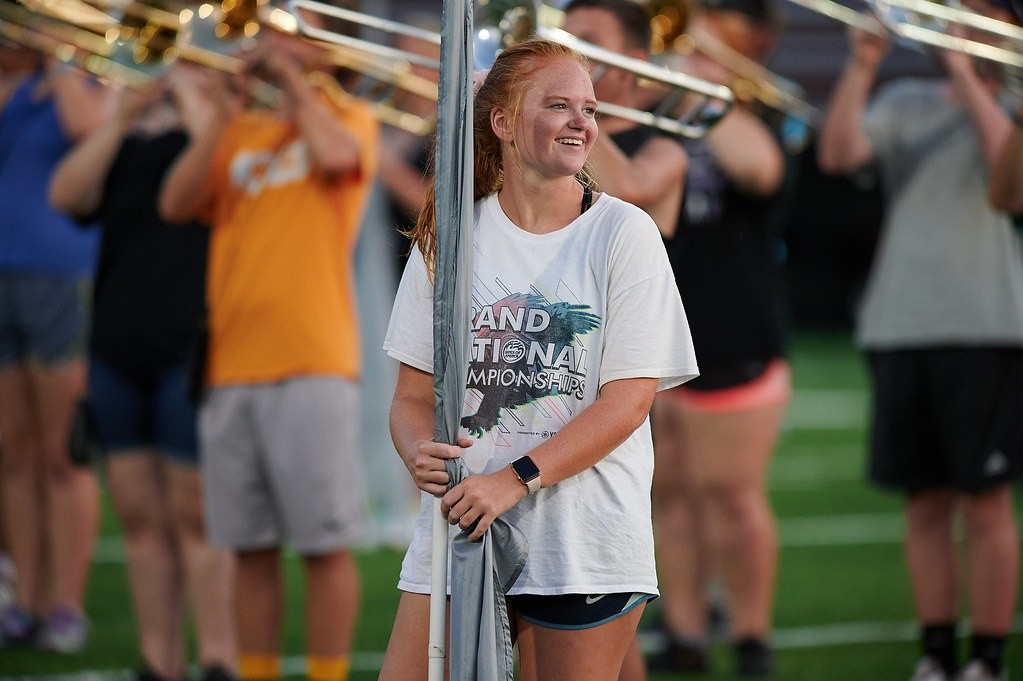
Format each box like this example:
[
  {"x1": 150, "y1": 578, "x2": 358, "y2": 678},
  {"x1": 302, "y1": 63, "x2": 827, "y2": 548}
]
[
  {"x1": 791, "y1": 0, "x2": 1023, "y2": 74},
  {"x1": 0, "y1": 0, "x2": 441, "y2": 139},
  {"x1": 281, "y1": 1, "x2": 737, "y2": 141}
]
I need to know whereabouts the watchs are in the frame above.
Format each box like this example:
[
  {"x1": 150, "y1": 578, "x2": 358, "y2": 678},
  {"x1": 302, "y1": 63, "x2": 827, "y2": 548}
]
[{"x1": 508, "y1": 455, "x2": 541, "y2": 496}]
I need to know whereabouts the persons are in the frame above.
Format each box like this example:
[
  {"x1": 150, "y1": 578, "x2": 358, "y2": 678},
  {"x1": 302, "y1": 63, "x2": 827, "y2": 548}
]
[
  {"x1": 384, "y1": 1, "x2": 1022, "y2": 681},
  {"x1": 0, "y1": 1, "x2": 472, "y2": 681},
  {"x1": 379, "y1": 40, "x2": 703, "y2": 681}
]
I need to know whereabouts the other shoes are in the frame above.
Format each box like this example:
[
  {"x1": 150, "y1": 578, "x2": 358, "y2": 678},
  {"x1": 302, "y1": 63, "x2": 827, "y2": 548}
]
[
  {"x1": 35, "y1": 606, "x2": 88, "y2": 653},
  {"x1": 0, "y1": 604, "x2": 36, "y2": 642},
  {"x1": 956, "y1": 660, "x2": 1008, "y2": 681},
  {"x1": 909, "y1": 656, "x2": 947, "y2": 681},
  {"x1": 733, "y1": 638, "x2": 769, "y2": 681},
  {"x1": 645, "y1": 633, "x2": 706, "y2": 676}
]
